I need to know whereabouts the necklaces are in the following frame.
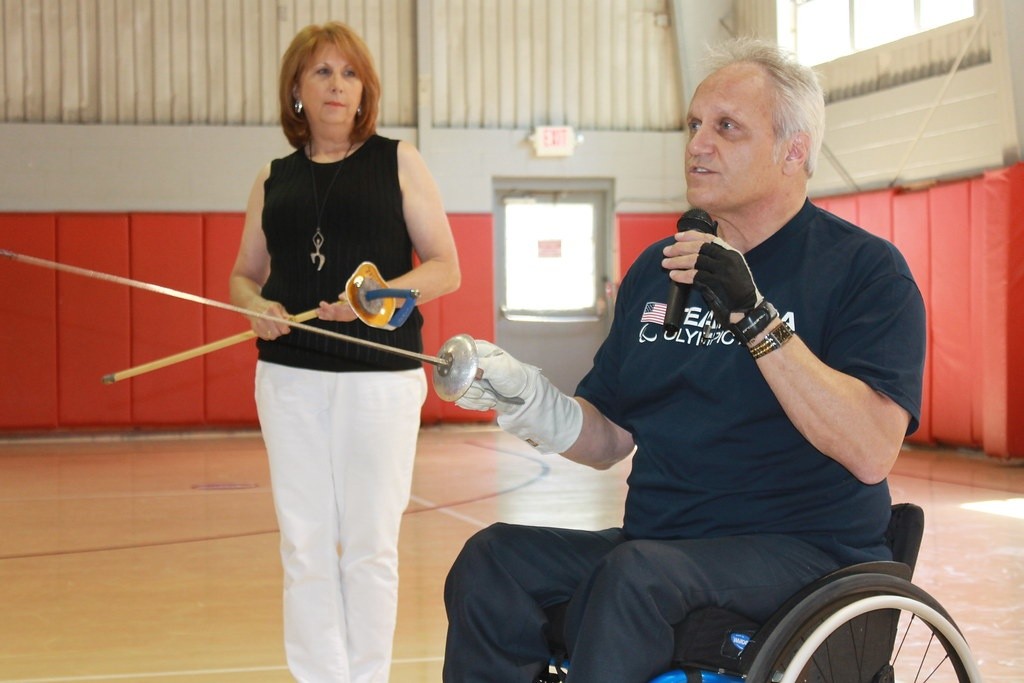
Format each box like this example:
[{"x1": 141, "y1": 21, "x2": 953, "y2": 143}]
[{"x1": 309, "y1": 139, "x2": 352, "y2": 270}]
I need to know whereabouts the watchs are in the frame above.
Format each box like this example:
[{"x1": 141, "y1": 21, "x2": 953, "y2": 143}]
[{"x1": 749, "y1": 323, "x2": 794, "y2": 358}]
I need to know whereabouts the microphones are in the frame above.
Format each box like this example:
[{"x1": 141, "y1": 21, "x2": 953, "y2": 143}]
[{"x1": 663, "y1": 209, "x2": 713, "y2": 332}]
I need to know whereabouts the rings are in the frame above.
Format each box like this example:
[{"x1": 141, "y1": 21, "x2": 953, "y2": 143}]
[{"x1": 266, "y1": 331, "x2": 271, "y2": 334}]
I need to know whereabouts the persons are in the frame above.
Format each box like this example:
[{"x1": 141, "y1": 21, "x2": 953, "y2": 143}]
[
  {"x1": 440, "y1": 30, "x2": 925, "y2": 683},
  {"x1": 228, "y1": 20, "x2": 461, "y2": 683}
]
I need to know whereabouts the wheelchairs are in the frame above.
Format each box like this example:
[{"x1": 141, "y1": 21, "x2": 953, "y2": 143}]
[{"x1": 530, "y1": 501, "x2": 983, "y2": 683}]
